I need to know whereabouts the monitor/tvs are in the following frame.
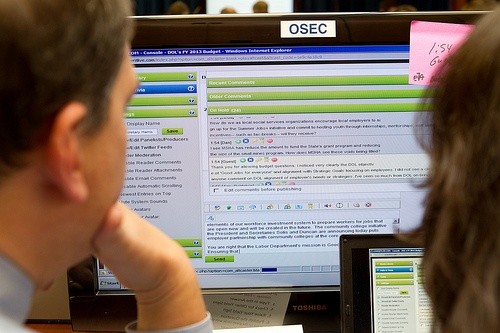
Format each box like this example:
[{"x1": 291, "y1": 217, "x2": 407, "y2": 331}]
[
  {"x1": 339, "y1": 233, "x2": 434, "y2": 333},
  {"x1": 59, "y1": 10, "x2": 500, "y2": 333}
]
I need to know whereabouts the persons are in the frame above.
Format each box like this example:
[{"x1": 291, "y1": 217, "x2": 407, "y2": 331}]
[
  {"x1": 389, "y1": 0, "x2": 500, "y2": 333},
  {"x1": 165, "y1": 2, "x2": 190, "y2": 15},
  {"x1": 252, "y1": 1, "x2": 269, "y2": 14},
  {"x1": 381, "y1": 3, "x2": 418, "y2": 12},
  {"x1": 0, "y1": 0, "x2": 217, "y2": 332},
  {"x1": 220, "y1": 8, "x2": 237, "y2": 15}
]
